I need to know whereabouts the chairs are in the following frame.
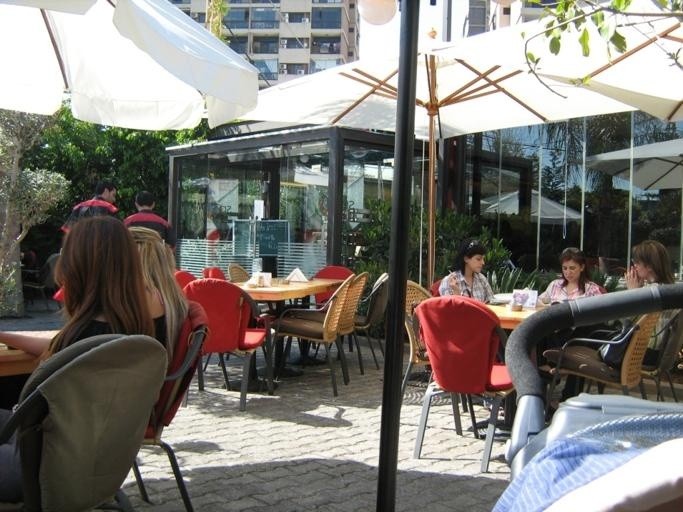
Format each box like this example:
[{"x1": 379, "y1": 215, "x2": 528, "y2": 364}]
[
  {"x1": 19, "y1": 253, "x2": 62, "y2": 311},
  {"x1": 131, "y1": 301, "x2": 209, "y2": 512},
  {"x1": 1, "y1": 332, "x2": 169, "y2": 511}
]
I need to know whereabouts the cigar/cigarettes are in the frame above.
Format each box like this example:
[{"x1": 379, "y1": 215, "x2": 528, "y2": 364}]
[{"x1": 448, "y1": 270, "x2": 456, "y2": 279}]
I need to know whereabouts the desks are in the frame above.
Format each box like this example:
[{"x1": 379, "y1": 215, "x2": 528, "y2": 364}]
[{"x1": 0, "y1": 330, "x2": 65, "y2": 377}]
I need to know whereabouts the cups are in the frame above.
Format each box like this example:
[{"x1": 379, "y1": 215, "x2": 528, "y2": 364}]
[
  {"x1": 510, "y1": 303, "x2": 523, "y2": 311},
  {"x1": 270, "y1": 278, "x2": 289, "y2": 287}
]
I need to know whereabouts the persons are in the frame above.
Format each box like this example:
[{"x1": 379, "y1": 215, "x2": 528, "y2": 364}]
[
  {"x1": 439, "y1": 239, "x2": 494, "y2": 304},
  {"x1": 1, "y1": 182, "x2": 187, "y2": 501},
  {"x1": 538, "y1": 246, "x2": 601, "y2": 303},
  {"x1": 549, "y1": 239, "x2": 677, "y2": 401}
]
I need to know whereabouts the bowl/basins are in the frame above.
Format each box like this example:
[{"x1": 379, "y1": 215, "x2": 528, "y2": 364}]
[{"x1": 489, "y1": 292, "x2": 514, "y2": 304}]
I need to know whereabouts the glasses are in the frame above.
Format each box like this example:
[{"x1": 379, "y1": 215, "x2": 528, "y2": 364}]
[{"x1": 466, "y1": 239, "x2": 481, "y2": 251}]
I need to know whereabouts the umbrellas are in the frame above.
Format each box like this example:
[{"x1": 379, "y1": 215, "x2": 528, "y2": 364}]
[
  {"x1": 0, "y1": 0, "x2": 259, "y2": 131},
  {"x1": 423, "y1": 0, "x2": 683, "y2": 122},
  {"x1": 584, "y1": 139, "x2": 683, "y2": 190},
  {"x1": 480, "y1": 188, "x2": 581, "y2": 224},
  {"x1": 239, "y1": 31, "x2": 638, "y2": 287}
]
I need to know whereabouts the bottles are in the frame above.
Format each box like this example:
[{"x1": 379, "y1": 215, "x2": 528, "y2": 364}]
[{"x1": 252, "y1": 258, "x2": 271, "y2": 289}]
